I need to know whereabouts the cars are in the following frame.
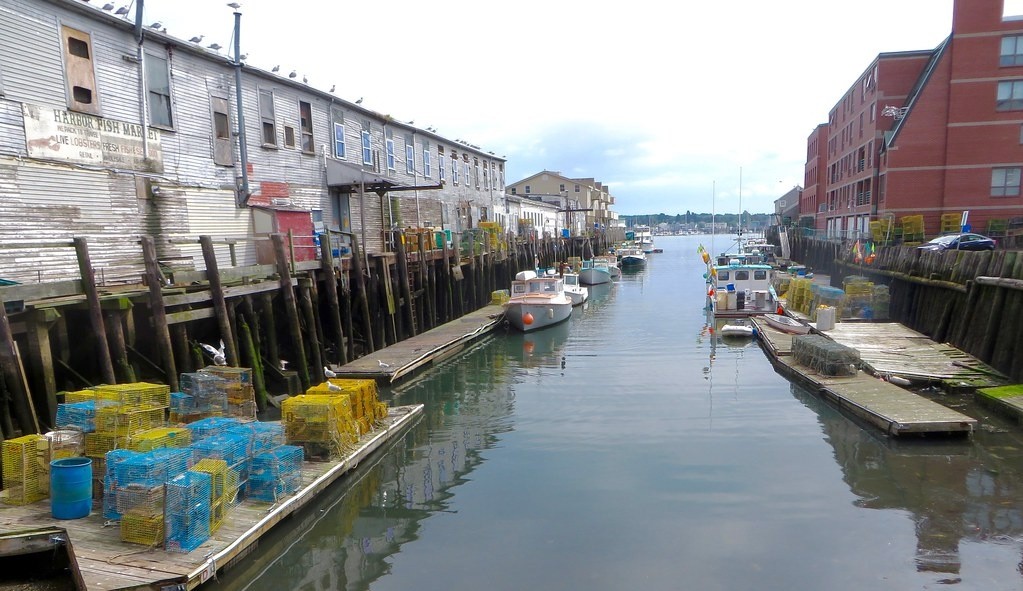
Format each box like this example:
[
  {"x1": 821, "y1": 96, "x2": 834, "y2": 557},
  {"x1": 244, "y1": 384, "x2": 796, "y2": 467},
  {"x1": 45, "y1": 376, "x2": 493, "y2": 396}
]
[{"x1": 916, "y1": 232, "x2": 997, "y2": 265}]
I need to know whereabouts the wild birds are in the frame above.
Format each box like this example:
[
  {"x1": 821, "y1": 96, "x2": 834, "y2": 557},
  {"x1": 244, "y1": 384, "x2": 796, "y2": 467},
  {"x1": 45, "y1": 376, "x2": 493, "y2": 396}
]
[
  {"x1": 378, "y1": 360, "x2": 389, "y2": 370},
  {"x1": 103, "y1": 1, "x2": 130, "y2": 15},
  {"x1": 148, "y1": 19, "x2": 163, "y2": 32},
  {"x1": 199, "y1": 338, "x2": 233, "y2": 366},
  {"x1": 189, "y1": 35, "x2": 506, "y2": 156},
  {"x1": 327, "y1": 381, "x2": 343, "y2": 392},
  {"x1": 323, "y1": 366, "x2": 337, "y2": 379}
]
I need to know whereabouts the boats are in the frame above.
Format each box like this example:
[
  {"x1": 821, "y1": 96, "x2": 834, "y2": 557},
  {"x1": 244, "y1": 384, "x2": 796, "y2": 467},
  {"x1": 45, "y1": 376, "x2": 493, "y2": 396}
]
[
  {"x1": 721, "y1": 324, "x2": 753, "y2": 337},
  {"x1": 787, "y1": 260, "x2": 806, "y2": 275},
  {"x1": 764, "y1": 314, "x2": 810, "y2": 335},
  {"x1": 706, "y1": 166, "x2": 782, "y2": 318},
  {"x1": 501, "y1": 277, "x2": 573, "y2": 335},
  {"x1": 543, "y1": 225, "x2": 663, "y2": 306},
  {"x1": 515, "y1": 271, "x2": 536, "y2": 282}
]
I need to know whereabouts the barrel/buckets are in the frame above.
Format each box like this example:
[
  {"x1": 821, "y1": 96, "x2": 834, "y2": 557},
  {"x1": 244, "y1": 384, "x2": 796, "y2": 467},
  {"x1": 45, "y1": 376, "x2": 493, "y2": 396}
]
[
  {"x1": 816, "y1": 308, "x2": 830, "y2": 331},
  {"x1": 826, "y1": 306, "x2": 836, "y2": 330},
  {"x1": 48, "y1": 456, "x2": 93, "y2": 520},
  {"x1": 727, "y1": 290, "x2": 737, "y2": 310},
  {"x1": 715, "y1": 289, "x2": 727, "y2": 310}
]
